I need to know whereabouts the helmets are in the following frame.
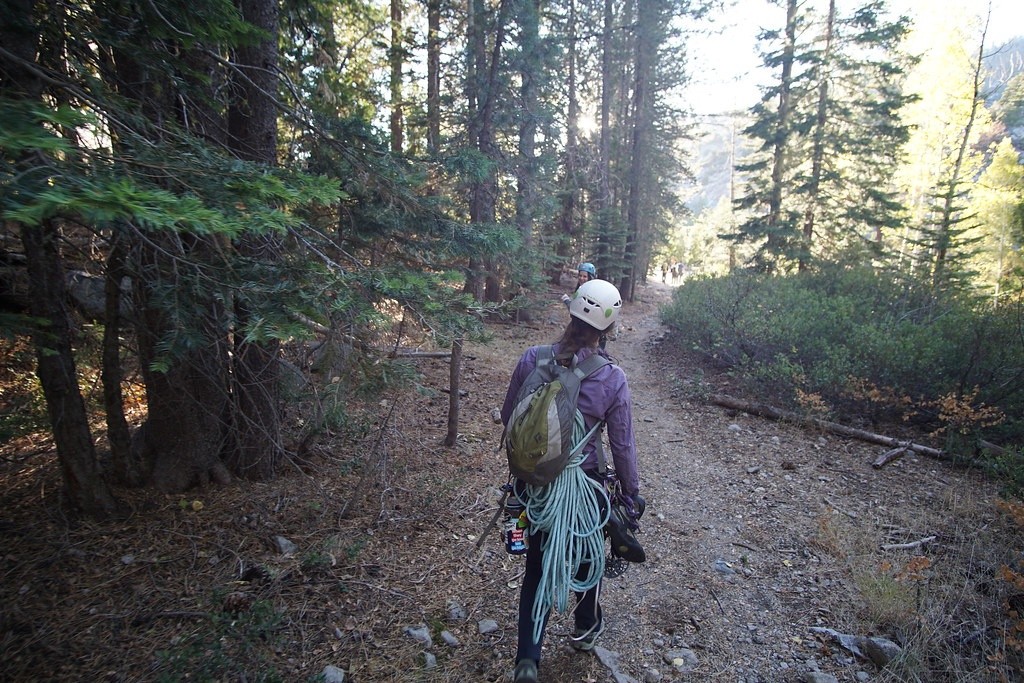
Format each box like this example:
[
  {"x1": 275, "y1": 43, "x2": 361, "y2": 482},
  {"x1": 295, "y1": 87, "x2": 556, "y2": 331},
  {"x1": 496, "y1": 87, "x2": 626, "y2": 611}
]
[
  {"x1": 570, "y1": 279, "x2": 622, "y2": 330},
  {"x1": 578, "y1": 262, "x2": 595, "y2": 277}
]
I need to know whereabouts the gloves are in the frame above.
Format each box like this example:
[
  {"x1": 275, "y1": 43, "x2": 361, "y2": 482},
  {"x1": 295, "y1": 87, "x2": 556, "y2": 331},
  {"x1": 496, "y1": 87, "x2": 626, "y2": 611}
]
[
  {"x1": 631, "y1": 495, "x2": 646, "y2": 521},
  {"x1": 561, "y1": 294, "x2": 569, "y2": 302}
]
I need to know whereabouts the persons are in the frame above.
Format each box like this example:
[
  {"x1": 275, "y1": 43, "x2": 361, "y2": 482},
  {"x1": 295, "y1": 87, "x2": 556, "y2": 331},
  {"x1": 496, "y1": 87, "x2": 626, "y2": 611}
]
[
  {"x1": 562, "y1": 263, "x2": 606, "y2": 350},
  {"x1": 661, "y1": 260, "x2": 684, "y2": 284},
  {"x1": 500, "y1": 279, "x2": 639, "y2": 683}
]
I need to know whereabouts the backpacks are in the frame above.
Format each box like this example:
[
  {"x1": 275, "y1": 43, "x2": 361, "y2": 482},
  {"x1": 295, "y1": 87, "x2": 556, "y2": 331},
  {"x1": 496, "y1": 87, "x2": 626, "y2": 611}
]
[{"x1": 506, "y1": 345, "x2": 613, "y2": 488}]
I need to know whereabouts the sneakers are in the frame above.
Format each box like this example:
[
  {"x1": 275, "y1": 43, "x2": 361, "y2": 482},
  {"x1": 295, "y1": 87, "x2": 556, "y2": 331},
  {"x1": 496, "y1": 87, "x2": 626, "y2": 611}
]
[
  {"x1": 514, "y1": 659, "x2": 537, "y2": 683},
  {"x1": 602, "y1": 505, "x2": 645, "y2": 563},
  {"x1": 573, "y1": 617, "x2": 605, "y2": 650}
]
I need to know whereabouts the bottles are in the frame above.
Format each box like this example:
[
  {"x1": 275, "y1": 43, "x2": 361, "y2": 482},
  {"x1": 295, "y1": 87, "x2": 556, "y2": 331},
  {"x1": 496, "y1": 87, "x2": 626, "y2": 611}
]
[{"x1": 505, "y1": 494, "x2": 529, "y2": 556}]
